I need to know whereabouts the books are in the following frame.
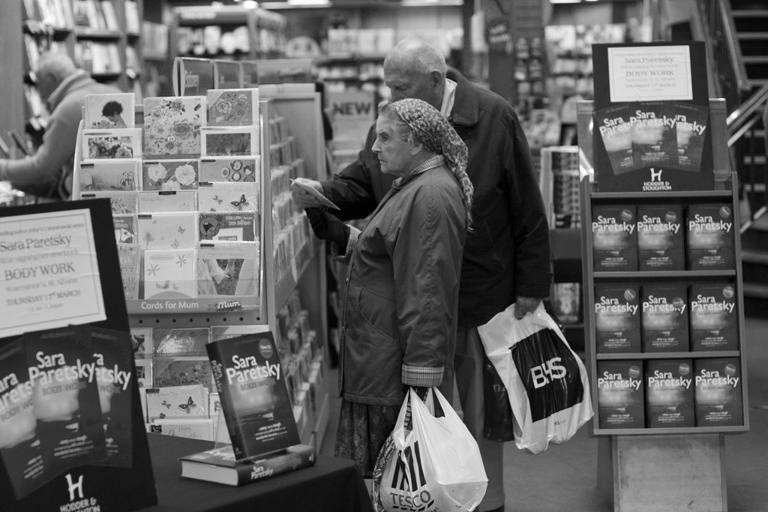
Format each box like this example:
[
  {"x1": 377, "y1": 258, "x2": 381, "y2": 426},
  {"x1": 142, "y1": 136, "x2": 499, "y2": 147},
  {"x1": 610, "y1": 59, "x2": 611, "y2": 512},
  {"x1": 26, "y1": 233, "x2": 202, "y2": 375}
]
[{"x1": 1, "y1": 5, "x2": 746, "y2": 510}]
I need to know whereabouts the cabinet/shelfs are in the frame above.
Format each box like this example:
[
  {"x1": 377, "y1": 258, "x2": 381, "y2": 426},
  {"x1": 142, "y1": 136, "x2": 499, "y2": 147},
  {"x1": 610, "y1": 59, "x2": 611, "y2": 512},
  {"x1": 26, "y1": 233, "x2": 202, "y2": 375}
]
[{"x1": 0, "y1": 0, "x2": 750, "y2": 457}]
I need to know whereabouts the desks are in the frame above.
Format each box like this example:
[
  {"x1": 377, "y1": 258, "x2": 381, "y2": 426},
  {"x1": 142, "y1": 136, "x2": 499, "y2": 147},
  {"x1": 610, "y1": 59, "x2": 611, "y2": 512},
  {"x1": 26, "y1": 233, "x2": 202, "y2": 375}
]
[{"x1": 136, "y1": 432, "x2": 375, "y2": 512}]
